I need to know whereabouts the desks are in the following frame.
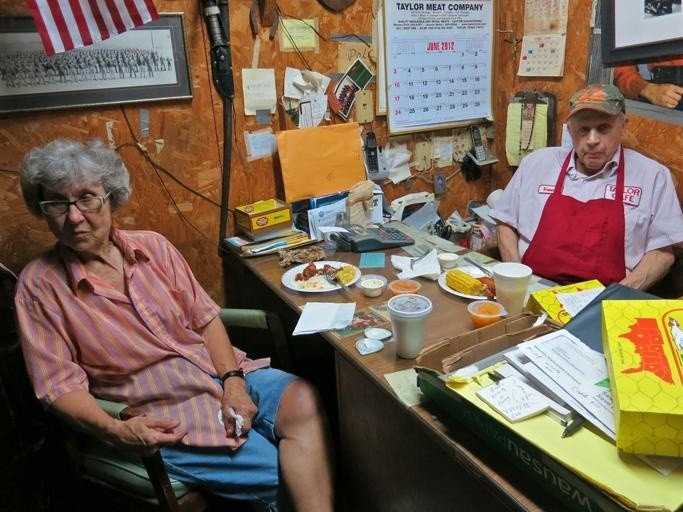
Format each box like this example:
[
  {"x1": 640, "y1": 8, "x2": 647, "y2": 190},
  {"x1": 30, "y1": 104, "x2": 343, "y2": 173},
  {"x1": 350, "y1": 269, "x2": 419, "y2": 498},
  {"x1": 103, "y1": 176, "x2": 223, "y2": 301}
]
[{"x1": 229, "y1": 219, "x2": 561, "y2": 512}]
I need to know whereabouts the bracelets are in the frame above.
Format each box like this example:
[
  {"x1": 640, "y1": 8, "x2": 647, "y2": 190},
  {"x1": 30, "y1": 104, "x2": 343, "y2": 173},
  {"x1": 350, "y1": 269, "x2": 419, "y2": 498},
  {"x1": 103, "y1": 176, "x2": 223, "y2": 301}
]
[{"x1": 221, "y1": 368, "x2": 247, "y2": 383}]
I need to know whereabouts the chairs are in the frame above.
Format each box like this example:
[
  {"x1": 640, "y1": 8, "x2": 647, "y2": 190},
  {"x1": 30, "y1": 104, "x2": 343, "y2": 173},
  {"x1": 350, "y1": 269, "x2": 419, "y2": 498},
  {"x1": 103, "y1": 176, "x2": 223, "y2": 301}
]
[{"x1": 13, "y1": 282, "x2": 288, "y2": 512}]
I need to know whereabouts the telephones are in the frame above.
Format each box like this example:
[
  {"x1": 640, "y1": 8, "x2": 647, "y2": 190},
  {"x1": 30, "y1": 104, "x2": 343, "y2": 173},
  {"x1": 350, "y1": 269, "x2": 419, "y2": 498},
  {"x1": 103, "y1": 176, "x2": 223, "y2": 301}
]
[
  {"x1": 364, "y1": 125, "x2": 389, "y2": 181},
  {"x1": 466, "y1": 125, "x2": 499, "y2": 167}
]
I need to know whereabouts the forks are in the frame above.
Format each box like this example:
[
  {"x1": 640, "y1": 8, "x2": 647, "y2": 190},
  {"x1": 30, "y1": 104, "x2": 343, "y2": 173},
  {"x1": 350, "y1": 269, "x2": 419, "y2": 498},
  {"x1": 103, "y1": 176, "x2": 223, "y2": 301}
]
[
  {"x1": 410, "y1": 248, "x2": 432, "y2": 271},
  {"x1": 324, "y1": 270, "x2": 352, "y2": 294}
]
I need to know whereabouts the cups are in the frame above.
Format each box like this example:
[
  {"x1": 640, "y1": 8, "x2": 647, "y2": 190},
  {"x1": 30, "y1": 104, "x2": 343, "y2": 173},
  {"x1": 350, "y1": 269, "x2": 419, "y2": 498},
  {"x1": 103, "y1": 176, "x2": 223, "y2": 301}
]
[
  {"x1": 386, "y1": 294, "x2": 432, "y2": 359},
  {"x1": 437, "y1": 253, "x2": 457, "y2": 267},
  {"x1": 362, "y1": 279, "x2": 384, "y2": 298},
  {"x1": 389, "y1": 279, "x2": 421, "y2": 296},
  {"x1": 466, "y1": 300, "x2": 505, "y2": 328},
  {"x1": 493, "y1": 263, "x2": 532, "y2": 318}
]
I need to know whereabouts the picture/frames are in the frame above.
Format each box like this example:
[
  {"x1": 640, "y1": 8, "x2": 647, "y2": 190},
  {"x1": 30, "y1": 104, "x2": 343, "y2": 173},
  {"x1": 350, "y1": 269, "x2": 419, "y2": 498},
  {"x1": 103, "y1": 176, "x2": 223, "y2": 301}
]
[
  {"x1": 0, "y1": 13, "x2": 195, "y2": 115},
  {"x1": 598, "y1": 0, "x2": 683, "y2": 68}
]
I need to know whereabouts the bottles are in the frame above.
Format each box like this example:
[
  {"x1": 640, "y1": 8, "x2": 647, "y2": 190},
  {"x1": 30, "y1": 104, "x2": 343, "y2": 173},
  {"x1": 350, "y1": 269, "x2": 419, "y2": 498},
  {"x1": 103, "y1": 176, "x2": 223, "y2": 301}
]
[{"x1": 336, "y1": 212, "x2": 347, "y2": 227}]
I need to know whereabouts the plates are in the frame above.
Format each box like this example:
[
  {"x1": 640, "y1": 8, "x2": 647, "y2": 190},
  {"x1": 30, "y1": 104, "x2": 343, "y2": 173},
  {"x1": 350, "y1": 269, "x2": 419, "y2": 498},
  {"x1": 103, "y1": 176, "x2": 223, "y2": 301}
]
[
  {"x1": 280, "y1": 260, "x2": 361, "y2": 295},
  {"x1": 438, "y1": 265, "x2": 495, "y2": 300}
]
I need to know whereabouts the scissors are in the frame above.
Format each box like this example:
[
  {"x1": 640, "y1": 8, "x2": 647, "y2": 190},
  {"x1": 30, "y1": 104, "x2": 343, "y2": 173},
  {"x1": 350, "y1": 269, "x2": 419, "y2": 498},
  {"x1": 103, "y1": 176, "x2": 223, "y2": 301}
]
[{"x1": 438, "y1": 225, "x2": 452, "y2": 240}]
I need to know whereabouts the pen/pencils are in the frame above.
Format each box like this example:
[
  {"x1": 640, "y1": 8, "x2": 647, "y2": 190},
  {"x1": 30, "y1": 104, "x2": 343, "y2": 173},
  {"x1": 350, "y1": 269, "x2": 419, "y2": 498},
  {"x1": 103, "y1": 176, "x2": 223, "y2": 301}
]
[
  {"x1": 488, "y1": 370, "x2": 504, "y2": 381},
  {"x1": 563, "y1": 416, "x2": 586, "y2": 438},
  {"x1": 547, "y1": 409, "x2": 568, "y2": 425}
]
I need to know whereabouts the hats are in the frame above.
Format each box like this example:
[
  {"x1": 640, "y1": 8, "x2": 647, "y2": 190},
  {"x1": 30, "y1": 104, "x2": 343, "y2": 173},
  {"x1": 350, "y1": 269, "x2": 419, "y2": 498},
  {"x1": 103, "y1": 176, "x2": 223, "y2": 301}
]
[{"x1": 566, "y1": 83, "x2": 625, "y2": 122}]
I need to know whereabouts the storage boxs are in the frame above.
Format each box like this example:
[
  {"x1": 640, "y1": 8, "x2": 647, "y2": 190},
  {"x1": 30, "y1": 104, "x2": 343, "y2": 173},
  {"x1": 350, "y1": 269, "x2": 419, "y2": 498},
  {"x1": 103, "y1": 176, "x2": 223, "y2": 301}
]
[
  {"x1": 413, "y1": 310, "x2": 682, "y2": 512},
  {"x1": 600, "y1": 299, "x2": 683, "y2": 458}
]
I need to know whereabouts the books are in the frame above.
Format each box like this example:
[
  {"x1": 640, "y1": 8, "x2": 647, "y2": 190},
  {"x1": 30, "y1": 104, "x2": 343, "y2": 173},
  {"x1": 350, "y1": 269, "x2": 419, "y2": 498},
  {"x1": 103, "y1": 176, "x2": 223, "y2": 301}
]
[
  {"x1": 472, "y1": 348, "x2": 578, "y2": 428},
  {"x1": 223, "y1": 227, "x2": 311, "y2": 258}
]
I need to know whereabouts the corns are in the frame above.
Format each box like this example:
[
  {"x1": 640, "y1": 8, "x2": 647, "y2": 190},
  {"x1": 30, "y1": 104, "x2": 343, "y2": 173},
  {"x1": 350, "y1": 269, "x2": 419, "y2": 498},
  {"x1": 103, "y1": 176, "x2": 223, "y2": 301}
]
[{"x1": 445, "y1": 269, "x2": 482, "y2": 297}]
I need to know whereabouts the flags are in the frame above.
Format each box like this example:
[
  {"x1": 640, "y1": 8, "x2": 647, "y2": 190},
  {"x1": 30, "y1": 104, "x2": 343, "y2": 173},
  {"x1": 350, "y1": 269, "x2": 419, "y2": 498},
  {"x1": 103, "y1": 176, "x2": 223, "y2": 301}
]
[{"x1": 26, "y1": 0, "x2": 160, "y2": 55}]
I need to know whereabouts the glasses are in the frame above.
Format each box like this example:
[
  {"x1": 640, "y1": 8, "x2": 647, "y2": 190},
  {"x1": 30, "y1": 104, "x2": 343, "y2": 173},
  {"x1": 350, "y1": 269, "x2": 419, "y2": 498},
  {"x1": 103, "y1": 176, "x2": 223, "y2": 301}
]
[{"x1": 38, "y1": 190, "x2": 111, "y2": 216}]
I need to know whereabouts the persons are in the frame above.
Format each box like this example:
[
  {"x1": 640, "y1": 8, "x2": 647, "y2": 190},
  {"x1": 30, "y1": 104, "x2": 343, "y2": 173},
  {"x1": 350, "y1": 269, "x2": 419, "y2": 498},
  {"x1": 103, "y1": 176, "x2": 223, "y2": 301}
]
[
  {"x1": 11, "y1": 138, "x2": 336, "y2": 512},
  {"x1": 335, "y1": 82, "x2": 357, "y2": 115},
  {"x1": 610, "y1": 58, "x2": 682, "y2": 113},
  {"x1": 488, "y1": 83, "x2": 682, "y2": 294},
  {"x1": 0, "y1": 47, "x2": 171, "y2": 89}
]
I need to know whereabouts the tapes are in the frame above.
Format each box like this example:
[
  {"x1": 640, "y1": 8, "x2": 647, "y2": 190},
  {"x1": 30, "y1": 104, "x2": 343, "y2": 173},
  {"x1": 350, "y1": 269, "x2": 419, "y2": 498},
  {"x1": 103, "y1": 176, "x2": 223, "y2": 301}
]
[{"x1": 445, "y1": 219, "x2": 471, "y2": 233}]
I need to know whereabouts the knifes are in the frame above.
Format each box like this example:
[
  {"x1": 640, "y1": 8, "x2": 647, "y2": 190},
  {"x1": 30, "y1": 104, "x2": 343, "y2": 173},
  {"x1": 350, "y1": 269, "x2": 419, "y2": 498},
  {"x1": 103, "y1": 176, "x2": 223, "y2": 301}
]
[{"x1": 463, "y1": 256, "x2": 493, "y2": 277}]
